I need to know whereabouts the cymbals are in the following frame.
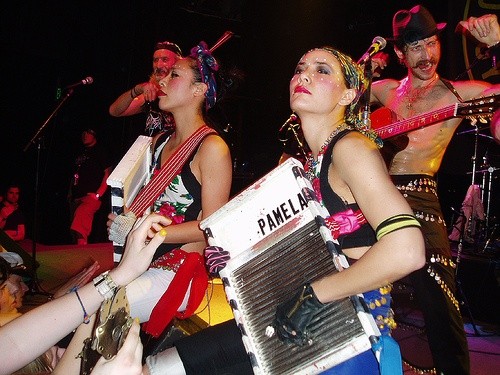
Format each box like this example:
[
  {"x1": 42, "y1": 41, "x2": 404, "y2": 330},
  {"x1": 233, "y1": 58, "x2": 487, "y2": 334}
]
[{"x1": 455, "y1": 125, "x2": 500, "y2": 174}]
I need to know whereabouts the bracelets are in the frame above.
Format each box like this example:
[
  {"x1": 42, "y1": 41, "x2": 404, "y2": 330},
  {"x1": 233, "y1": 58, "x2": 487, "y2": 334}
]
[
  {"x1": 92, "y1": 272, "x2": 116, "y2": 300},
  {"x1": 71, "y1": 286, "x2": 92, "y2": 325},
  {"x1": 374, "y1": 213, "x2": 423, "y2": 243},
  {"x1": 130, "y1": 84, "x2": 142, "y2": 99}
]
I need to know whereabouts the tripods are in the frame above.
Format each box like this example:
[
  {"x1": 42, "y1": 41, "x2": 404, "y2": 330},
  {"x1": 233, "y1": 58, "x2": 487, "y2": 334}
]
[{"x1": 24, "y1": 90, "x2": 76, "y2": 298}]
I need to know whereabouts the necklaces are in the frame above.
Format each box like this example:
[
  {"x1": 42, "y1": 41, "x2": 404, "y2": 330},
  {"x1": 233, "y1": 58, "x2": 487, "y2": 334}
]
[
  {"x1": 403, "y1": 72, "x2": 439, "y2": 110},
  {"x1": 304, "y1": 118, "x2": 346, "y2": 179}
]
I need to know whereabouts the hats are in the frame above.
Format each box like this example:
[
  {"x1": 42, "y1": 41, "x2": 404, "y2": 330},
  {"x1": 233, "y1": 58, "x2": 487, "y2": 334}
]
[
  {"x1": 0, "y1": 255, "x2": 8, "y2": 286},
  {"x1": 85, "y1": 127, "x2": 97, "y2": 137},
  {"x1": 0, "y1": 252, "x2": 31, "y2": 277},
  {"x1": 384, "y1": 4, "x2": 448, "y2": 42}
]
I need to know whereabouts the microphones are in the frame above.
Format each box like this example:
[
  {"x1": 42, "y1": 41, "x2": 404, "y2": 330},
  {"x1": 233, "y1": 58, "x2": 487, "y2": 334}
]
[
  {"x1": 356, "y1": 35, "x2": 387, "y2": 66},
  {"x1": 279, "y1": 114, "x2": 298, "y2": 132},
  {"x1": 66, "y1": 76, "x2": 94, "y2": 90}
]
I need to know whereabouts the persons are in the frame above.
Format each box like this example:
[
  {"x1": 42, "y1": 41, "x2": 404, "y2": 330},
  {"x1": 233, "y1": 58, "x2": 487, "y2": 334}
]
[
  {"x1": 1, "y1": 250, "x2": 30, "y2": 312},
  {"x1": 450, "y1": 182, "x2": 487, "y2": 251},
  {"x1": 202, "y1": 45, "x2": 427, "y2": 375},
  {"x1": 52, "y1": 49, "x2": 232, "y2": 374},
  {"x1": 110, "y1": 39, "x2": 185, "y2": 139},
  {"x1": 0, "y1": 212, "x2": 175, "y2": 374},
  {"x1": 0, "y1": 185, "x2": 26, "y2": 243},
  {"x1": 71, "y1": 124, "x2": 112, "y2": 247},
  {"x1": 363, "y1": 6, "x2": 500, "y2": 374},
  {"x1": 1, "y1": 260, "x2": 108, "y2": 374}
]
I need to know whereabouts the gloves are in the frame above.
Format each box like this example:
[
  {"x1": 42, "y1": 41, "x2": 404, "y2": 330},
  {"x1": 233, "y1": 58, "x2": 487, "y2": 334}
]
[
  {"x1": 203, "y1": 246, "x2": 230, "y2": 277},
  {"x1": 274, "y1": 282, "x2": 324, "y2": 348}
]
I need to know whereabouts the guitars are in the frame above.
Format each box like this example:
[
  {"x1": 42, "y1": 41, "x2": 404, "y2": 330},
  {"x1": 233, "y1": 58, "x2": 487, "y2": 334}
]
[{"x1": 373, "y1": 94, "x2": 500, "y2": 140}]
